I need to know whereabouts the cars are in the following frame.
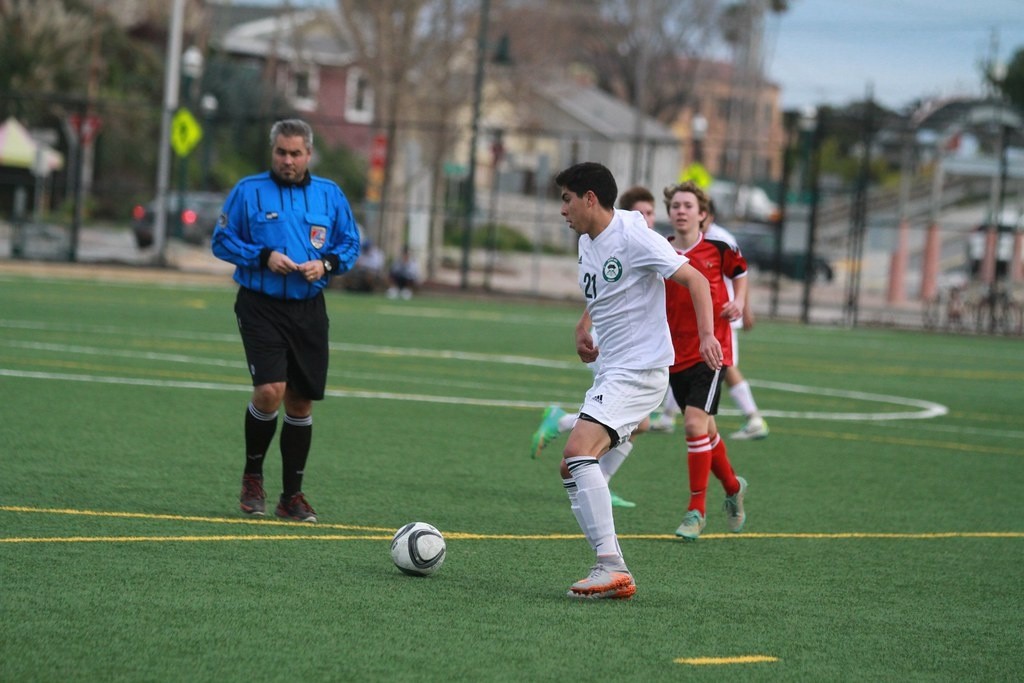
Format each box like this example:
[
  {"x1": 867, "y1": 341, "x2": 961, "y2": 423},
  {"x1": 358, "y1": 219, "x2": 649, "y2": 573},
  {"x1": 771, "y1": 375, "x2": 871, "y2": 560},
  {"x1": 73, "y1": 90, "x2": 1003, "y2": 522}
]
[{"x1": 132, "y1": 187, "x2": 223, "y2": 248}]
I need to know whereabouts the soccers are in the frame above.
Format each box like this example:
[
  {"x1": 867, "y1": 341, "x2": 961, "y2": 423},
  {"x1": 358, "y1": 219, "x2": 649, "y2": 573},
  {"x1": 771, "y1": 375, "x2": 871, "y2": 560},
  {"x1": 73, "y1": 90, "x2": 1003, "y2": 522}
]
[{"x1": 390, "y1": 521, "x2": 447, "y2": 577}]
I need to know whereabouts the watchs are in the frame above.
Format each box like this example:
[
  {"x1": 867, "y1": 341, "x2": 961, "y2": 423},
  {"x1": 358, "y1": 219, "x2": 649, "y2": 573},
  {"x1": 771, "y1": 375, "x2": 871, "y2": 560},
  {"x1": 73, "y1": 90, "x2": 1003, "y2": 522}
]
[{"x1": 322, "y1": 258, "x2": 332, "y2": 273}]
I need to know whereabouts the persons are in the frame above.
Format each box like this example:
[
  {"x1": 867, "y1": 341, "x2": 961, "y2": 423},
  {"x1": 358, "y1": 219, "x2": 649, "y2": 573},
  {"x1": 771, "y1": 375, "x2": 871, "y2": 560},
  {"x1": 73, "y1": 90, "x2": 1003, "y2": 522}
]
[
  {"x1": 387, "y1": 243, "x2": 422, "y2": 301},
  {"x1": 211, "y1": 119, "x2": 360, "y2": 522},
  {"x1": 360, "y1": 237, "x2": 386, "y2": 290},
  {"x1": 530, "y1": 184, "x2": 769, "y2": 541},
  {"x1": 554, "y1": 162, "x2": 724, "y2": 599}
]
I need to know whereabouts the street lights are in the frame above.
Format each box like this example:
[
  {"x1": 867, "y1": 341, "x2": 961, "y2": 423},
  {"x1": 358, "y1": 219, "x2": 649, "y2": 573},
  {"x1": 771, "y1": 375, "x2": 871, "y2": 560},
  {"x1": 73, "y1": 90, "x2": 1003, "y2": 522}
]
[{"x1": 461, "y1": 17, "x2": 516, "y2": 288}]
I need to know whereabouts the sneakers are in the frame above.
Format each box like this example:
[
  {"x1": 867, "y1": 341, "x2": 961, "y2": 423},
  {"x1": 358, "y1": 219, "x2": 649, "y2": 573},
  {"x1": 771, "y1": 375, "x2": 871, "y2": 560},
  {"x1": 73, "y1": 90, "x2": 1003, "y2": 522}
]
[
  {"x1": 240, "y1": 474, "x2": 267, "y2": 515},
  {"x1": 729, "y1": 419, "x2": 769, "y2": 439},
  {"x1": 566, "y1": 562, "x2": 636, "y2": 599},
  {"x1": 725, "y1": 475, "x2": 747, "y2": 532},
  {"x1": 275, "y1": 491, "x2": 317, "y2": 523},
  {"x1": 674, "y1": 507, "x2": 707, "y2": 540},
  {"x1": 611, "y1": 492, "x2": 636, "y2": 508},
  {"x1": 649, "y1": 416, "x2": 675, "y2": 434},
  {"x1": 531, "y1": 405, "x2": 566, "y2": 460}
]
